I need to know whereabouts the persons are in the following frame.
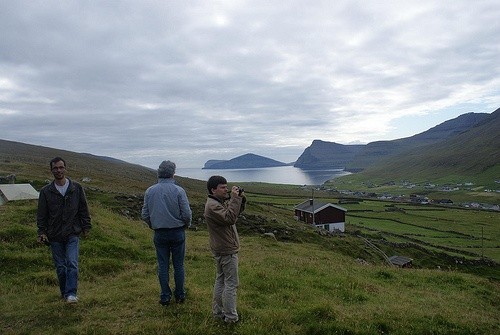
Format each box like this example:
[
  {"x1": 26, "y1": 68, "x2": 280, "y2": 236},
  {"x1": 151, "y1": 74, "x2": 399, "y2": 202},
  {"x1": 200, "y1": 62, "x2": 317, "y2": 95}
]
[
  {"x1": 142, "y1": 161, "x2": 192, "y2": 305},
  {"x1": 203, "y1": 176, "x2": 247, "y2": 324},
  {"x1": 37, "y1": 156, "x2": 92, "y2": 305}
]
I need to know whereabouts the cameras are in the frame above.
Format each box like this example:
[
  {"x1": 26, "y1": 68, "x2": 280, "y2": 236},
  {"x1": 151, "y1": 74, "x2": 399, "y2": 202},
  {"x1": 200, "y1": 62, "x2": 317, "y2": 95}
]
[
  {"x1": 236, "y1": 188, "x2": 244, "y2": 197},
  {"x1": 40, "y1": 238, "x2": 51, "y2": 247}
]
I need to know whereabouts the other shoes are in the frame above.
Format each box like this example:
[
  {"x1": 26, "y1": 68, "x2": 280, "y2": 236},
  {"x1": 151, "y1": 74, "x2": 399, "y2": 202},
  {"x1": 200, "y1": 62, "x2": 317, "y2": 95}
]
[
  {"x1": 223, "y1": 315, "x2": 241, "y2": 329},
  {"x1": 66, "y1": 294, "x2": 78, "y2": 304},
  {"x1": 176, "y1": 296, "x2": 186, "y2": 303},
  {"x1": 159, "y1": 296, "x2": 172, "y2": 307}
]
